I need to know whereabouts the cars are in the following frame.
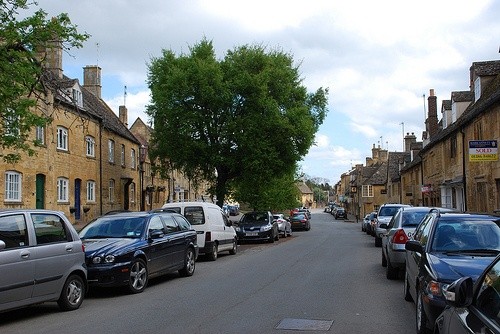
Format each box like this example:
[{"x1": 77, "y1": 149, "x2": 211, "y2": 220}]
[
  {"x1": 361, "y1": 211, "x2": 378, "y2": 237},
  {"x1": 429, "y1": 250, "x2": 500, "y2": 334},
  {"x1": 378, "y1": 207, "x2": 456, "y2": 280},
  {"x1": 324, "y1": 204, "x2": 348, "y2": 220},
  {"x1": 273, "y1": 213, "x2": 292, "y2": 238},
  {"x1": 287, "y1": 208, "x2": 312, "y2": 231},
  {"x1": 234, "y1": 211, "x2": 280, "y2": 244},
  {"x1": 221, "y1": 205, "x2": 240, "y2": 218}
]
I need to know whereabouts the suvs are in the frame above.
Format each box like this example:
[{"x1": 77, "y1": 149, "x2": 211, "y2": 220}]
[
  {"x1": 75, "y1": 208, "x2": 199, "y2": 294},
  {"x1": 401, "y1": 208, "x2": 500, "y2": 334},
  {"x1": 373, "y1": 204, "x2": 414, "y2": 247},
  {"x1": 0, "y1": 209, "x2": 89, "y2": 315}
]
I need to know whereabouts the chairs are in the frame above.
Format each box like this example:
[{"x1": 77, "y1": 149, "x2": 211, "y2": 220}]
[
  {"x1": 404, "y1": 214, "x2": 418, "y2": 224},
  {"x1": 110, "y1": 224, "x2": 123, "y2": 234},
  {"x1": 385, "y1": 209, "x2": 392, "y2": 215},
  {"x1": 436, "y1": 225, "x2": 458, "y2": 246}
]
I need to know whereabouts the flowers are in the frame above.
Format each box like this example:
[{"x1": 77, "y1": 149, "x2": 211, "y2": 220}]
[
  {"x1": 145, "y1": 184, "x2": 157, "y2": 193},
  {"x1": 158, "y1": 186, "x2": 167, "y2": 192}
]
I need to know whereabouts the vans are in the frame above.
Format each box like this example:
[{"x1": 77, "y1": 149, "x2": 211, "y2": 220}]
[{"x1": 159, "y1": 202, "x2": 239, "y2": 260}]
